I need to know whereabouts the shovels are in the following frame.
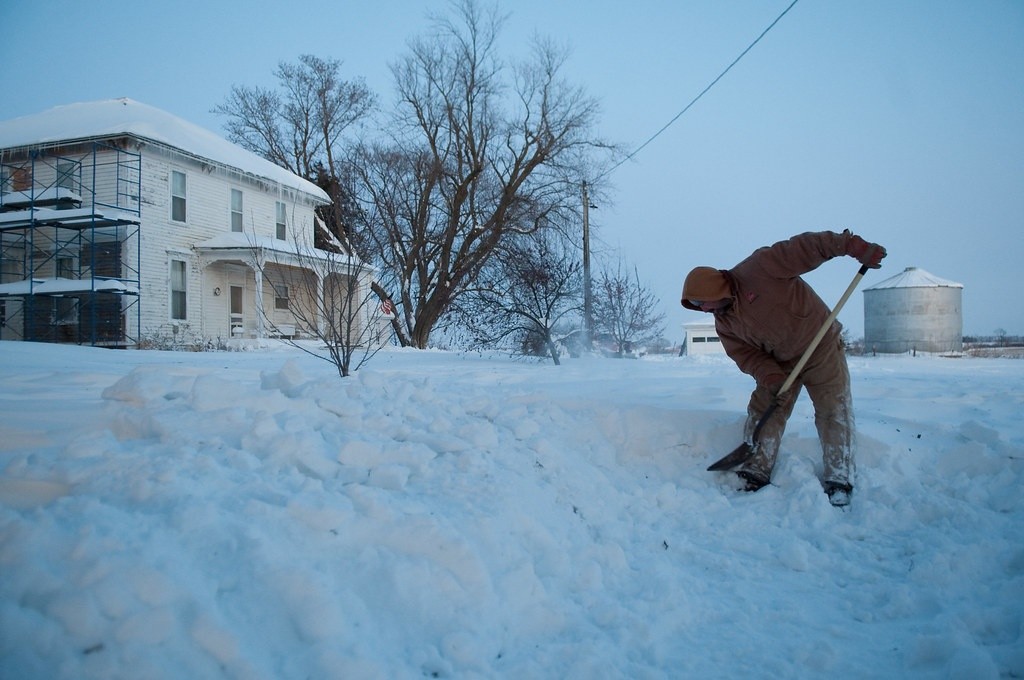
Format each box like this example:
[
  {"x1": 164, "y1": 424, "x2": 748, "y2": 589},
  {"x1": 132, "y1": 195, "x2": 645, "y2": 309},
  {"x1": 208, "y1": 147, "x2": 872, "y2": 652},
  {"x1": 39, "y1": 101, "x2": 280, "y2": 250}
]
[{"x1": 706, "y1": 263, "x2": 870, "y2": 473}]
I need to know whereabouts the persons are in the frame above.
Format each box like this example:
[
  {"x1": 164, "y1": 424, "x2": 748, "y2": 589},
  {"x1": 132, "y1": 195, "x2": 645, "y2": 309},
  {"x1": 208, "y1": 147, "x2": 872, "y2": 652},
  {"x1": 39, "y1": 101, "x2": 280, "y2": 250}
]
[{"x1": 681, "y1": 228, "x2": 888, "y2": 506}]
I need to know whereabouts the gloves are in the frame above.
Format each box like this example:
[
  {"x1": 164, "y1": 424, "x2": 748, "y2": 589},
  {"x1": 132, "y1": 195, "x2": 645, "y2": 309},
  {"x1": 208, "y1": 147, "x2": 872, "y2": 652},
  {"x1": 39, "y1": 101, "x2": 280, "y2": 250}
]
[
  {"x1": 846, "y1": 235, "x2": 887, "y2": 269},
  {"x1": 765, "y1": 374, "x2": 794, "y2": 407}
]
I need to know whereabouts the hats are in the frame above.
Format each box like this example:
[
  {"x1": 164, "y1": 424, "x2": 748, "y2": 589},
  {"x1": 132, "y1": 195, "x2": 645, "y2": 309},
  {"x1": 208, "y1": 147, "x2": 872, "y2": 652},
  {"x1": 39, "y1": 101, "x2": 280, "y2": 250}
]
[{"x1": 688, "y1": 299, "x2": 704, "y2": 306}]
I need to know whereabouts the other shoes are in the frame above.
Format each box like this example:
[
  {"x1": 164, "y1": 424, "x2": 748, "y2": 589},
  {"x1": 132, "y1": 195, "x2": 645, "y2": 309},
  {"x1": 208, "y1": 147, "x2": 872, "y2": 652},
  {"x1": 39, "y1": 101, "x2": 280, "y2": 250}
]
[
  {"x1": 734, "y1": 471, "x2": 771, "y2": 492},
  {"x1": 823, "y1": 481, "x2": 853, "y2": 507}
]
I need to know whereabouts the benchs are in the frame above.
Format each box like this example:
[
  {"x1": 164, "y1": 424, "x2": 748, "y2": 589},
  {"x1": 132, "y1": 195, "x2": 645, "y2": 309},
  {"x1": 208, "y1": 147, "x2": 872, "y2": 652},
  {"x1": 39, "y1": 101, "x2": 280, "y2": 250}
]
[{"x1": 272, "y1": 324, "x2": 296, "y2": 340}]
